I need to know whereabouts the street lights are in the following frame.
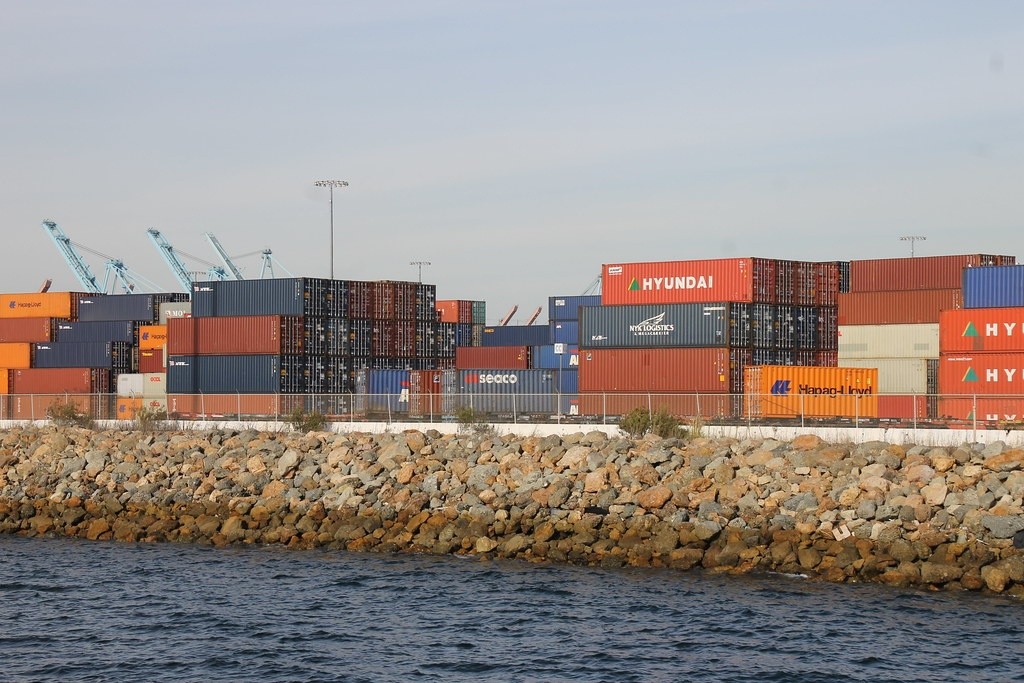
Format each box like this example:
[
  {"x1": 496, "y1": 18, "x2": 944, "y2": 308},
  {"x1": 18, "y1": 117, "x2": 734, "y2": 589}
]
[
  {"x1": 900, "y1": 236, "x2": 929, "y2": 259},
  {"x1": 410, "y1": 261, "x2": 431, "y2": 284},
  {"x1": 315, "y1": 180, "x2": 349, "y2": 280}
]
